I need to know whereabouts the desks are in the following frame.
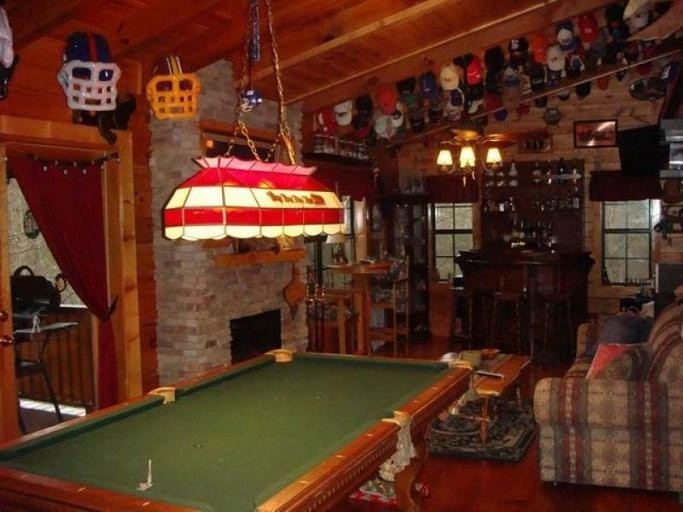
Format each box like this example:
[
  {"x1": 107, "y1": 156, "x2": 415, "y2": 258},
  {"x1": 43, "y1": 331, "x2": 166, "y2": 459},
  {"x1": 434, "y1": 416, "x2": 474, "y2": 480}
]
[
  {"x1": 1, "y1": 349, "x2": 472, "y2": 512},
  {"x1": 454, "y1": 247, "x2": 595, "y2": 364}
]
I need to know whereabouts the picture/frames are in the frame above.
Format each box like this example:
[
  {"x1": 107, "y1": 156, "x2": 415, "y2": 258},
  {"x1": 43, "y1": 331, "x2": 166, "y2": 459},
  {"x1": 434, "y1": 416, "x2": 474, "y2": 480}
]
[
  {"x1": 573, "y1": 118, "x2": 618, "y2": 149},
  {"x1": 23, "y1": 209, "x2": 40, "y2": 239}
]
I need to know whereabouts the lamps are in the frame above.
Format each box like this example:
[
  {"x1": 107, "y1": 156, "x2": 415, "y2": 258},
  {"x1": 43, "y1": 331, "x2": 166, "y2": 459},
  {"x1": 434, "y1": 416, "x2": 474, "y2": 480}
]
[
  {"x1": 436, "y1": 127, "x2": 510, "y2": 186},
  {"x1": 161, "y1": 0, "x2": 347, "y2": 241}
]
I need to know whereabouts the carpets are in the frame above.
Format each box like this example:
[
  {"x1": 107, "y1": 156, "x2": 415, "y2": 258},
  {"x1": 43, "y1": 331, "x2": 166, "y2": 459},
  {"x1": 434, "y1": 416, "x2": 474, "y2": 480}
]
[{"x1": 424, "y1": 389, "x2": 537, "y2": 466}]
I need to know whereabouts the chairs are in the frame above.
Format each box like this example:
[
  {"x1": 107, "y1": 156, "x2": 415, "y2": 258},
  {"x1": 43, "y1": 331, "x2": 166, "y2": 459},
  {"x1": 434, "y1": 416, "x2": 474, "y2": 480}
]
[{"x1": 9, "y1": 265, "x2": 80, "y2": 434}]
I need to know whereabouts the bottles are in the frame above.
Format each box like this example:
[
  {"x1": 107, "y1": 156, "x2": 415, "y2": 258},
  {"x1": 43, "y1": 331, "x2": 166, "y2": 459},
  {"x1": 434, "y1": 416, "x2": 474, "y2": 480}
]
[{"x1": 478, "y1": 156, "x2": 584, "y2": 260}]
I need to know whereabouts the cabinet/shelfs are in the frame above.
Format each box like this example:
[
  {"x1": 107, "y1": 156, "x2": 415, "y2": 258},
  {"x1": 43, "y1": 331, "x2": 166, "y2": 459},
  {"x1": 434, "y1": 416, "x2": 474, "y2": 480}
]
[
  {"x1": 366, "y1": 190, "x2": 433, "y2": 343},
  {"x1": 369, "y1": 256, "x2": 410, "y2": 356},
  {"x1": 480, "y1": 158, "x2": 583, "y2": 249}
]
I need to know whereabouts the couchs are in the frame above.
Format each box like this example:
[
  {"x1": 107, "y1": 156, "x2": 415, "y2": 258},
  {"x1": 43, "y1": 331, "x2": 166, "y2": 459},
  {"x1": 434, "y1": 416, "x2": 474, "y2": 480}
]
[{"x1": 533, "y1": 287, "x2": 682, "y2": 504}]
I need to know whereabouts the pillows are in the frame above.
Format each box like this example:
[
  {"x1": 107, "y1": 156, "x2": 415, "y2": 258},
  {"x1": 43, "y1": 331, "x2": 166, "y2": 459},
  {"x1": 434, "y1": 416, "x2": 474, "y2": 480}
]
[
  {"x1": 587, "y1": 315, "x2": 653, "y2": 360},
  {"x1": 587, "y1": 343, "x2": 652, "y2": 381}
]
